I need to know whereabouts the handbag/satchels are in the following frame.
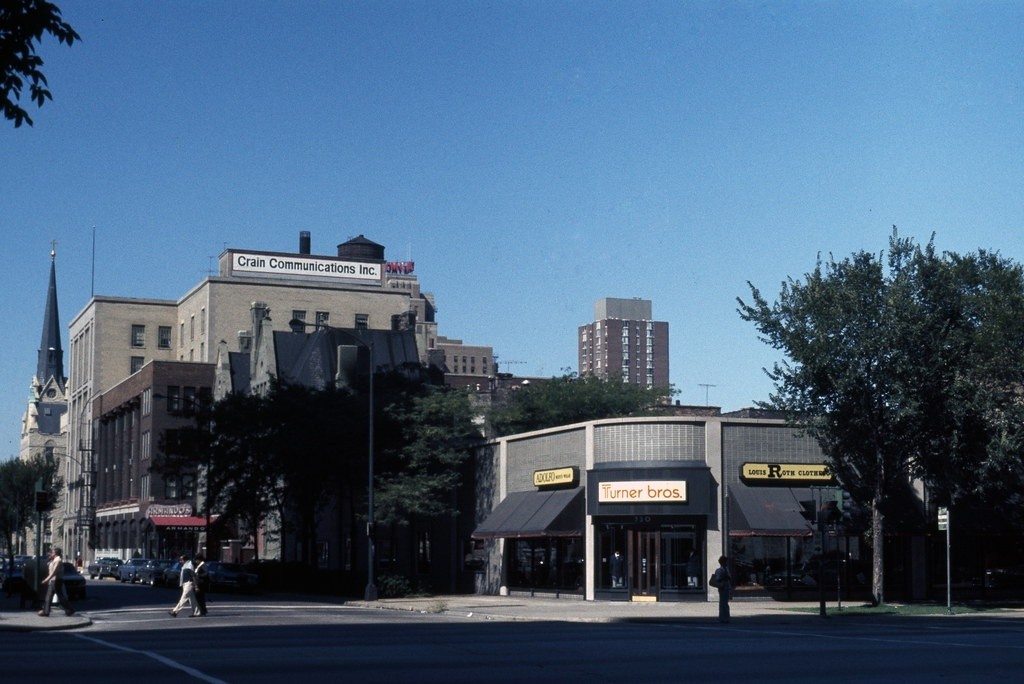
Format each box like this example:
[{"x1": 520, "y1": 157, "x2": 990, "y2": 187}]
[{"x1": 709, "y1": 568, "x2": 726, "y2": 589}]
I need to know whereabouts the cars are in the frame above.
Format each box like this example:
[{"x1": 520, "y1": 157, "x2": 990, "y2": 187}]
[
  {"x1": 88, "y1": 558, "x2": 123, "y2": 581},
  {"x1": 0, "y1": 555, "x2": 48, "y2": 604},
  {"x1": 117, "y1": 558, "x2": 149, "y2": 583},
  {"x1": 205, "y1": 562, "x2": 258, "y2": 594},
  {"x1": 59, "y1": 562, "x2": 86, "y2": 598},
  {"x1": 138, "y1": 560, "x2": 163, "y2": 588},
  {"x1": 162, "y1": 561, "x2": 183, "y2": 589}
]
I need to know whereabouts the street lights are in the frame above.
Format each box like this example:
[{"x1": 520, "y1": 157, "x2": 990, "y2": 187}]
[
  {"x1": 152, "y1": 392, "x2": 212, "y2": 561},
  {"x1": 45, "y1": 450, "x2": 84, "y2": 561},
  {"x1": 289, "y1": 318, "x2": 379, "y2": 601}
]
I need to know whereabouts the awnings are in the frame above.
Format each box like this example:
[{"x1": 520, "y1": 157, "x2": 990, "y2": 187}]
[{"x1": 469, "y1": 486, "x2": 585, "y2": 537}]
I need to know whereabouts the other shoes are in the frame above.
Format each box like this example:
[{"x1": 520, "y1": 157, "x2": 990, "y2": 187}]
[
  {"x1": 167, "y1": 611, "x2": 177, "y2": 617},
  {"x1": 66, "y1": 610, "x2": 74, "y2": 615},
  {"x1": 38, "y1": 612, "x2": 49, "y2": 616},
  {"x1": 190, "y1": 615, "x2": 196, "y2": 617}
]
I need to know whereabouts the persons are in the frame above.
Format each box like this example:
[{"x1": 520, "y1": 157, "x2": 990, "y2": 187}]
[
  {"x1": 191, "y1": 554, "x2": 209, "y2": 617},
  {"x1": 77, "y1": 555, "x2": 84, "y2": 575},
  {"x1": 37, "y1": 547, "x2": 76, "y2": 617},
  {"x1": 686, "y1": 548, "x2": 701, "y2": 587},
  {"x1": 715, "y1": 556, "x2": 733, "y2": 624},
  {"x1": 169, "y1": 554, "x2": 202, "y2": 618},
  {"x1": 610, "y1": 550, "x2": 625, "y2": 588}
]
